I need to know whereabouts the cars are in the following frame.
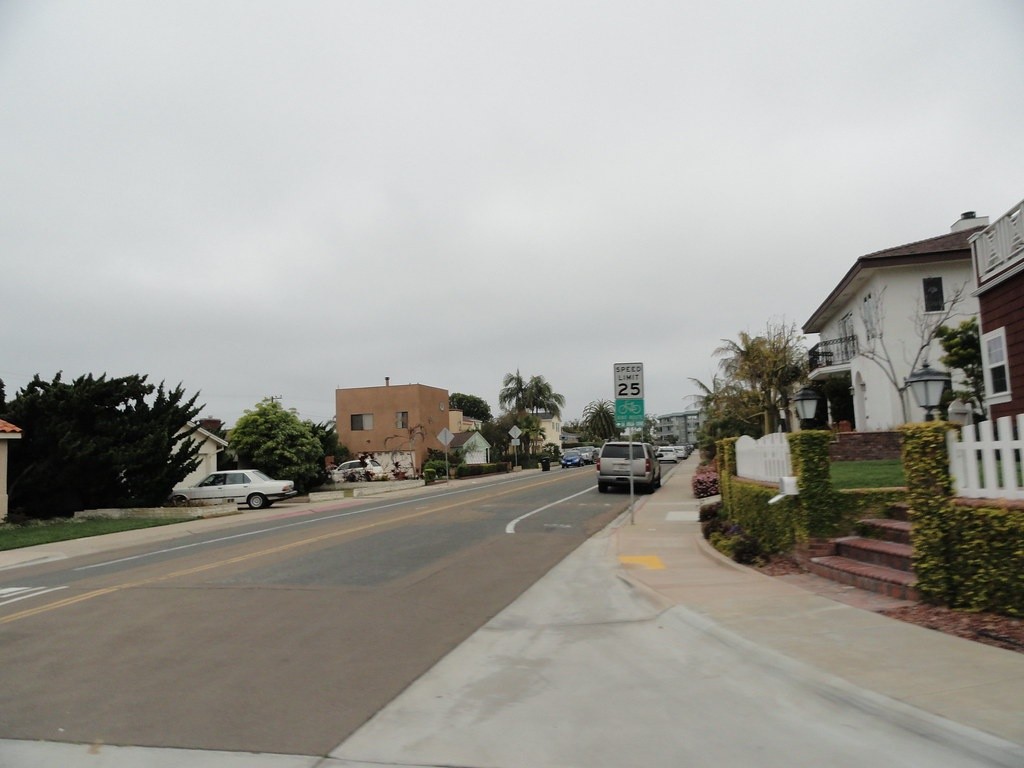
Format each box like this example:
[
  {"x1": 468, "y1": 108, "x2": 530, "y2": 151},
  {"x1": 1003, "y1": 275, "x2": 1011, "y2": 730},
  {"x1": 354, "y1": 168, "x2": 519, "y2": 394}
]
[
  {"x1": 656, "y1": 447, "x2": 678, "y2": 463},
  {"x1": 327, "y1": 459, "x2": 383, "y2": 482},
  {"x1": 562, "y1": 450, "x2": 584, "y2": 468},
  {"x1": 674, "y1": 445, "x2": 694, "y2": 459},
  {"x1": 168, "y1": 469, "x2": 298, "y2": 509}
]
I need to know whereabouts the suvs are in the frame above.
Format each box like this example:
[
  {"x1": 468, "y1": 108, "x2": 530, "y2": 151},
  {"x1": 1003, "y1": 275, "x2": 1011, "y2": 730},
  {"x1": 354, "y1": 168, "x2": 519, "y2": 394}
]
[
  {"x1": 571, "y1": 446, "x2": 599, "y2": 465},
  {"x1": 596, "y1": 442, "x2": 664, "y2": 494}
]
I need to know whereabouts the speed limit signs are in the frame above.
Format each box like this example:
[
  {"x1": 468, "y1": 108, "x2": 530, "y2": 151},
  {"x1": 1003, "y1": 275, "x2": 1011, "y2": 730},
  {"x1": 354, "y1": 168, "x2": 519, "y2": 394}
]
[{"x1": 613, "y1": 362, "x2": 644, "y2": 398}]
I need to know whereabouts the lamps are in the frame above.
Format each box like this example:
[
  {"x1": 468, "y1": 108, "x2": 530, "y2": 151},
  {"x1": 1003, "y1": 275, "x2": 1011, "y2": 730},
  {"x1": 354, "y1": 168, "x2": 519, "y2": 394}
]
[
  {"x1": 904, "y1": 358, "x2": 952, "y2": 414},
  {"x1": 860, "y1": 382, "x2": 865, "y2": 391},
  {"x1": 777, "y1": 405, "x2": 787, "y2": 419},
  {"x1": 848, "y1": 386, "x2": 854, "y2": 395},
  {"x1": 791, "y1": 384, "x2": 822, "y2": 429}
]
